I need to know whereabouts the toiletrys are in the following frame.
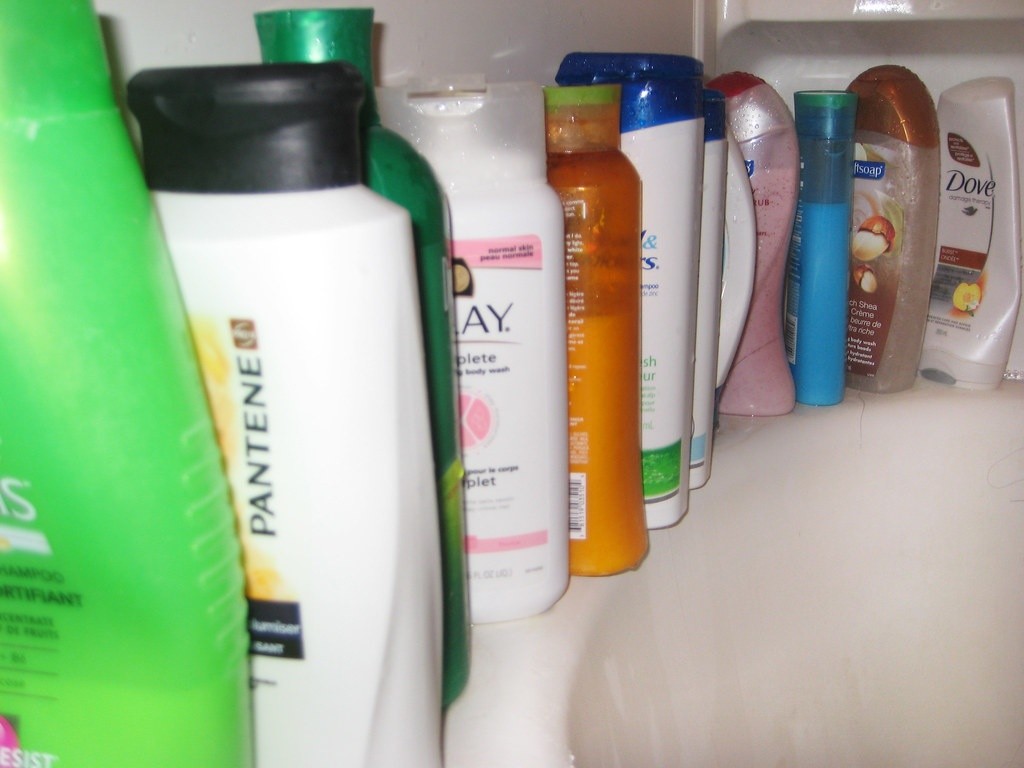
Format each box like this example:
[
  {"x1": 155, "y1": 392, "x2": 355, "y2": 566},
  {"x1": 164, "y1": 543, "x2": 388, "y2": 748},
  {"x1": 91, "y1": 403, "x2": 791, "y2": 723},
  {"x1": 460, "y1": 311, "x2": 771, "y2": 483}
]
[{"x1": 0, "y1": 1, "x2": 1024, "y2": 768}]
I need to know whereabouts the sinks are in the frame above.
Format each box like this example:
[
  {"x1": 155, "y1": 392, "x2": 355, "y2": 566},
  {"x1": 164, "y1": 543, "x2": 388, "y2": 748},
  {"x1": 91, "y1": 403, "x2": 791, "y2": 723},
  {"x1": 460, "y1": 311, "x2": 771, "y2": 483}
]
[{"x1": 562, "y1": 406, "x2": 1024, "y2": 767}]
